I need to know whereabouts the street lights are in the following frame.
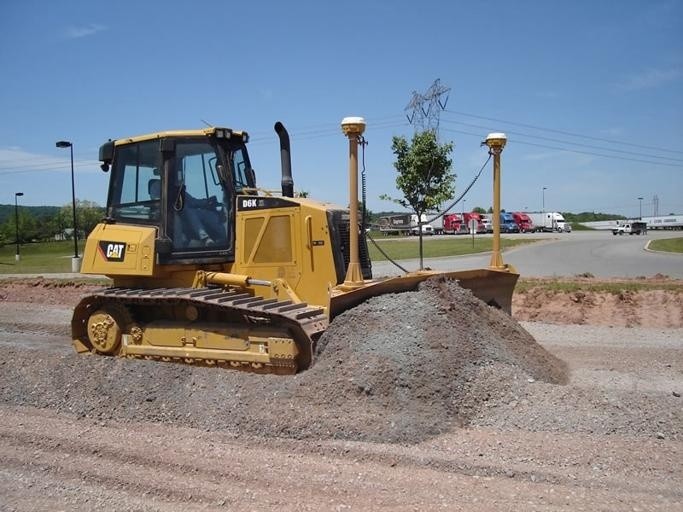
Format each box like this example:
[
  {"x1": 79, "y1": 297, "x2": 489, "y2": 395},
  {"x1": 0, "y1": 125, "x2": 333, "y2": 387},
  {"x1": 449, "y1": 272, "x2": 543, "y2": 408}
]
[
  {"x1": 14, "y1": 191, "x2": 25, "y2": 260},
  {"x1": 541, "y1": 187, "x2": 548, "y2": 210},
  {"x1": 638, "y1": 197, "x2": 643, "y2": 218},
  {"x1": 55, "y1": 140, "x2": 82, "y2": 274},
  {"x1": 461, "y1": 198, "x2": 466, "y2": 211}
]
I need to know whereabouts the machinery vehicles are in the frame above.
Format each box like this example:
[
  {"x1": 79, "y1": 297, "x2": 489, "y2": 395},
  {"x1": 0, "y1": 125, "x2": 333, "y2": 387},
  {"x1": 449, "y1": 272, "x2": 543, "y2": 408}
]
[{"x1": 66, "y1": 115, "x2": 518, "y2": 375}]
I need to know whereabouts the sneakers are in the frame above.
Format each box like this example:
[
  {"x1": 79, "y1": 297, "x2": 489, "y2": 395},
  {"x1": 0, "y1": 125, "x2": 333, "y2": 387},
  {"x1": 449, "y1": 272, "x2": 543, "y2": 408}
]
[{"x1": 204, "y1": 238, "x2": 215, "y2": 248}]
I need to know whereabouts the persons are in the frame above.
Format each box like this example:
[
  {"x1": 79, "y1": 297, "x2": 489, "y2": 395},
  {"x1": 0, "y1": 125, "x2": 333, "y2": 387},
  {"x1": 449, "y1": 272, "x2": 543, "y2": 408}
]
[{"x1": 152, "y1": 165, "x2": 225, "y2": 247}]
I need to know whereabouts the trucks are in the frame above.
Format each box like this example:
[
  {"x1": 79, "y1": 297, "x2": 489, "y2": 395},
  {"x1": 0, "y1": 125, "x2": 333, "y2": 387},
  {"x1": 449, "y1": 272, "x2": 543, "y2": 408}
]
[
  {"x1": 377, "y1": 210, "x2": 571, "y2": 235},
  {"x1": 610, "y1": 221, "x2": 645, "y2": 235},
  {"x1": 639, "y1": 214, "x2": 683, "y2": 231}
]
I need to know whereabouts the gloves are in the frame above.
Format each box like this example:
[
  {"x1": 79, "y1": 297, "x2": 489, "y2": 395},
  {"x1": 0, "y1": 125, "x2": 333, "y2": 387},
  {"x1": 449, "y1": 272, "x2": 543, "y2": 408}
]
[{"x1": 207, "y1": 196, "x2": 218, "y2": 206}]
